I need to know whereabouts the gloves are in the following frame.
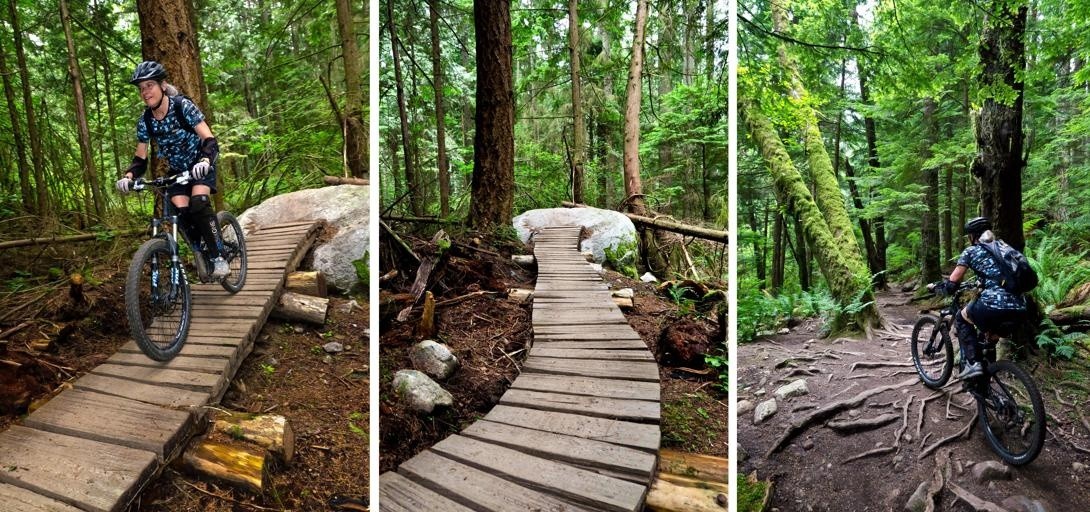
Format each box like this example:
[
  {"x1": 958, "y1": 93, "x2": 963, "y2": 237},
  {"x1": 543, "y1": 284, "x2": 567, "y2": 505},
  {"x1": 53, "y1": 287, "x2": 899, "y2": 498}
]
[
  {"x1": 116, "y1": 177, "x2": 131, "y2": 194},
  {"x1": 192, "y1": 160, "x2": 210, "y2": 181}
]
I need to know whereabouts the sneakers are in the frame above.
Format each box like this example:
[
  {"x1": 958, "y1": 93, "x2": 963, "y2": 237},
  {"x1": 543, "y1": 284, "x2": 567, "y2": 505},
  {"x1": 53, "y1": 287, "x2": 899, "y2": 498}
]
[
  {"x1": 953, "y1": 360, "x2": 983, "y2": 382},
  {"x1": 211, "y1": 256, "x2": 232, "y2": 280}
]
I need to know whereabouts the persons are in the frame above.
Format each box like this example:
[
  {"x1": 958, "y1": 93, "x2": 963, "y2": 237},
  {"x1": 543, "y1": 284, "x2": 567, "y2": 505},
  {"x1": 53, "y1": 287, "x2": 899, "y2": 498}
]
[
  {"x1": 115, "y1": 60, "x2": 232, "y2": 279},
  {"x1": 925, "y1": 217, "x2": 1039, "y2": 380}
]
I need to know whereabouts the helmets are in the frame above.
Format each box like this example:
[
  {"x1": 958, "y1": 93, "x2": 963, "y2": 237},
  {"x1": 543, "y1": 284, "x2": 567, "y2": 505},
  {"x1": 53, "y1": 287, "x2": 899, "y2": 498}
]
[
  {"x1": 961, "y1": 217, "x2": 993, "y2": 236},
  {"x1": 128, "y1": 60, "x2": 168, "y2": 86}
]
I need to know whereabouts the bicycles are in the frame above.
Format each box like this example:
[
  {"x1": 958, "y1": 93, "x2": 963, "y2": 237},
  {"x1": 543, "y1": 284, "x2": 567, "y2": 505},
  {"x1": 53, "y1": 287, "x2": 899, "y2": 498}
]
[
  {"x1": 123, "y1": 165, "x2": 249, "y2": 363},
  {"x1": 911, "y1": 281, "x2": 1047, "y2": 467}
]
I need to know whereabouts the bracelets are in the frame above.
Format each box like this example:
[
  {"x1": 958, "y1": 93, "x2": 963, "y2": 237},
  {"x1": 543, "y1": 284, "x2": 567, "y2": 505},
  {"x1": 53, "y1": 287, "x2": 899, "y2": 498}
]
[
  {"x1": 124, "y1": 176, "x2": 131, "y2": 179},
  {"x1": 931, "y1": 284, "x2": 934, "y2": 288}
]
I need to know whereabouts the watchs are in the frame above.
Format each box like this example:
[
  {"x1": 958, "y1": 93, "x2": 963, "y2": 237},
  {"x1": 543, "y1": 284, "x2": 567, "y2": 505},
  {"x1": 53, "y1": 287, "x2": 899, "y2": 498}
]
[{"x1": 200, "y1": 159, "x2": 210, "y2": 164}]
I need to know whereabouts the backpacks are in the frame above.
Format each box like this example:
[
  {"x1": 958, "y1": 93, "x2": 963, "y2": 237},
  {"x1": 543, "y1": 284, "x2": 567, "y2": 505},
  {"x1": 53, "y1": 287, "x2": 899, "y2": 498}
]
[{"x1": 973, "y1": 240, "x2": 1039, "y2": 294}]
